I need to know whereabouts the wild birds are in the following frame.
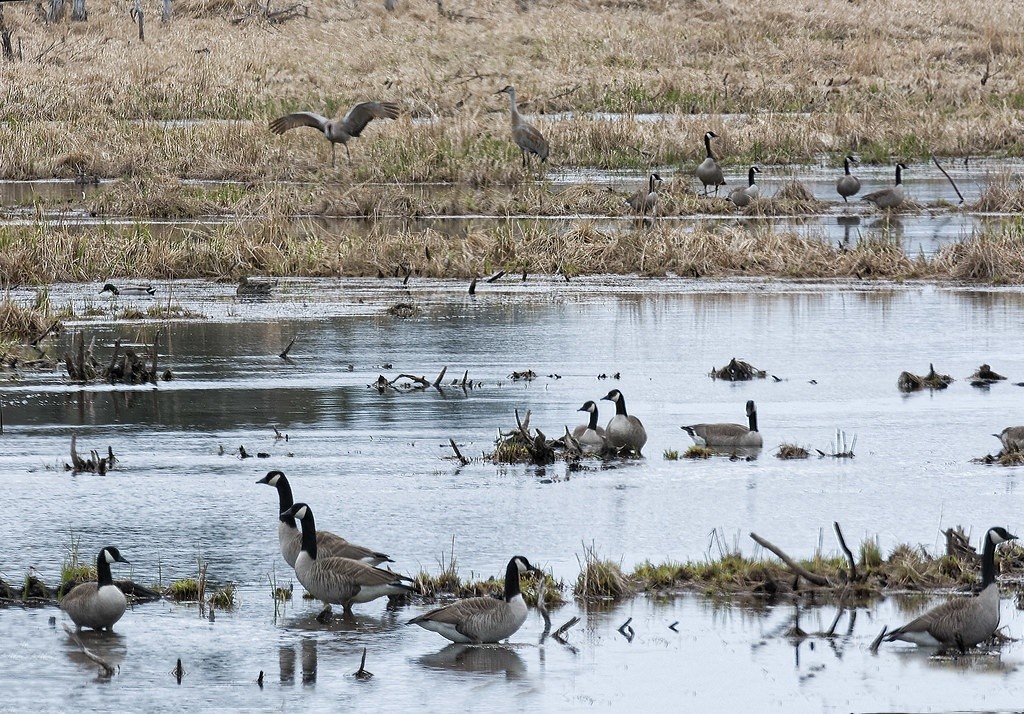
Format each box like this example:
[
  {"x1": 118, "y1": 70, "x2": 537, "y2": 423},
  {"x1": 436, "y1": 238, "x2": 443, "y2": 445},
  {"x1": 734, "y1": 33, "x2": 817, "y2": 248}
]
[
  {"x1": 626, "y1": 174, "x2": 663, "y2": 215},
  {"x1": 279, "y1": 502, "x2": 419, "y2": 616},
  {"x1": 680, "y1": 401, "x2": 763, "y2": 448},
  {"x1": 994, "y1": 426, "x2": 1024, "y2": 453},
  {"x1": 726, "y1": 167, "x2": 763, "y2": 209},
  {"x1": 860, "y1": 163, "x2": 910, "y2": 210},
  {"x1": 234, "y1": 276, "x2": 272, "y2": 295},
  {"x1": 494, "y1": 85, "x2": 550, "y2": 166},
  {"x1": 61, "y1": 546, "x2": 131, "y2": 632},
  {"x1": 572, "y1": 401, "x2": 607, "y2": 456},
  {"x1": 836, "y1": 156, "x2": 861, "y2": 203},
  {"x1": 268, "y1": 101, "x2": 400, "y2": 167},
  {"x1": 404, "y1": 555, "x2": 543, "y2": 645},
  {"x1": 696, "y1": 132, "x2": 727, "y2": 198},
  {"x1": 99, "y1": 284, "x2": 156, "y2": 295},
  {"x1": 880, "y1": 527, "x2": 1018, "y2": 651},
  {"x1": 255, "y1": 470, "x2": 397, "y2": 568},
  {"x1": 599, "y1": 389, "x2": 647, "y2": 452}
]
